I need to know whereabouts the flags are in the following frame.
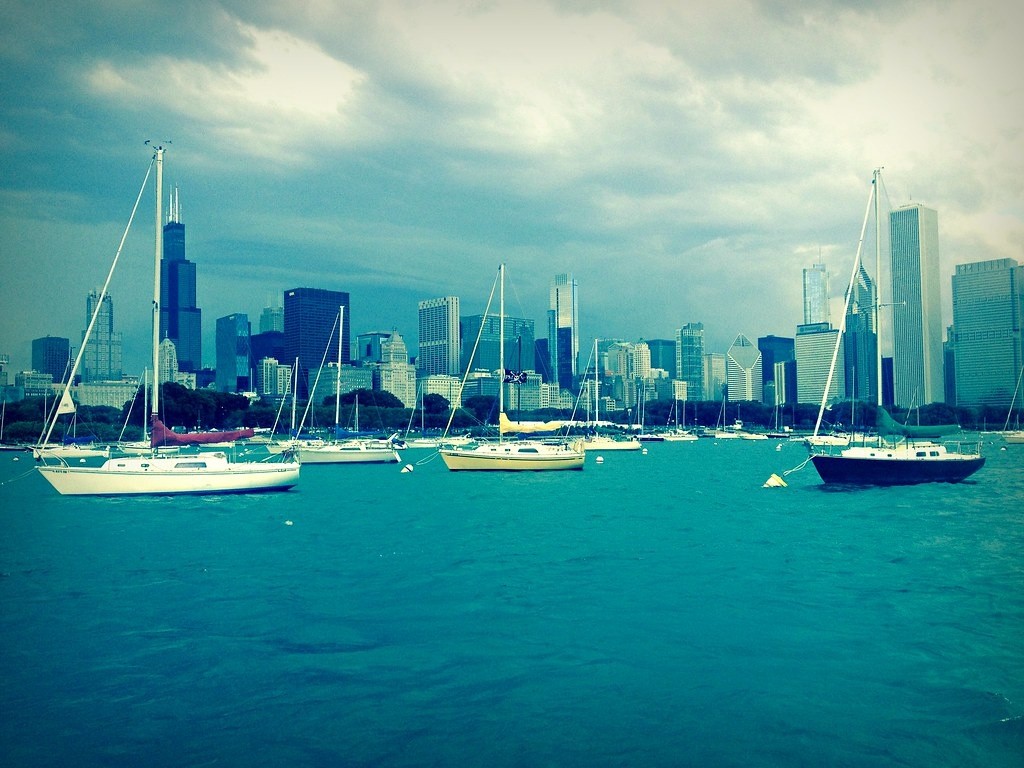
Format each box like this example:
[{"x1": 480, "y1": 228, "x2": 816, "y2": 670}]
[{"x1": 502, "y1": 369, "x2": 527, "y2": 384}]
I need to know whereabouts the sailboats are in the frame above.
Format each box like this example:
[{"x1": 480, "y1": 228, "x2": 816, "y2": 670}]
[
  {"x1": 282, "y1": 302, "x2": 404, "y2": 466},
  {"x1": 804, "y1": 164, "x2": 987, "y2": 488},
  {"x1": 1000, "y1": 362, "x2": 1024, "y2": 446},
  {"x1": 0, "y1": 338, "x2": 884, "y2": 468},
  {"x1": 32, "y1": 144, "x2": 301, "y2": 497},
  {"x1": 435, "y1": 261, "x2": 588, "y2": 472}
]
